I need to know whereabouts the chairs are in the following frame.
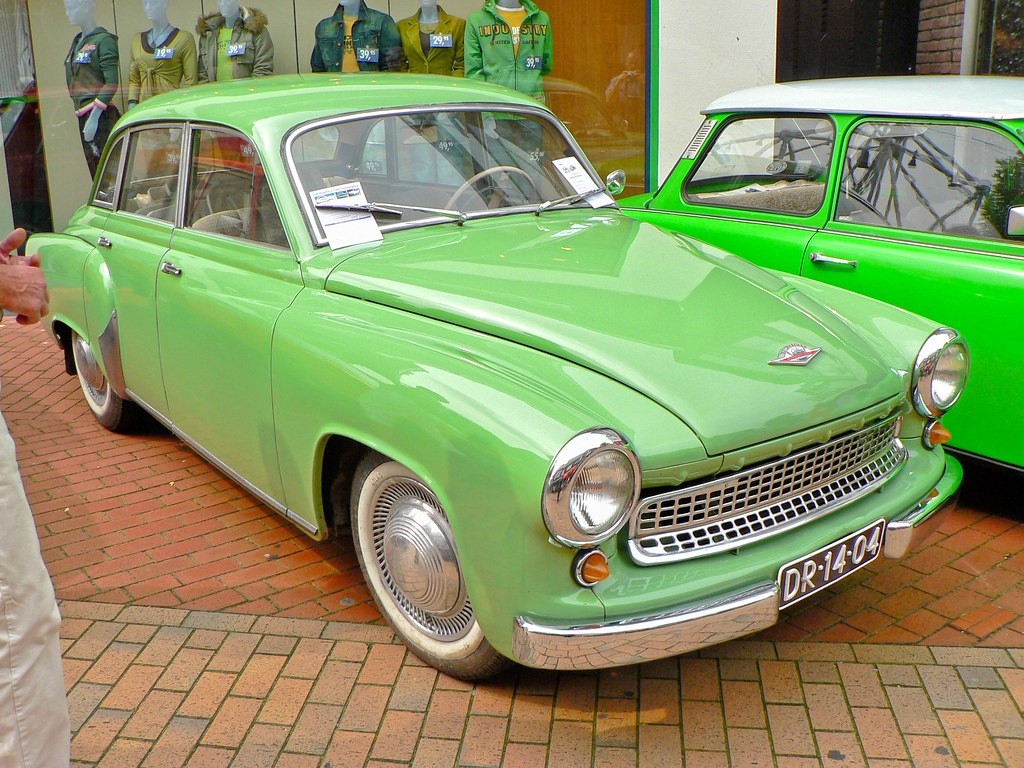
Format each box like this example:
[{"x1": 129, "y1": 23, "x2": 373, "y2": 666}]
[
  {"x1": 908, "y1": 200, "x2": 1001, "y2": 240},
  {"x1": 192, "y1": 200, "x2": 291, "y2": 249}
]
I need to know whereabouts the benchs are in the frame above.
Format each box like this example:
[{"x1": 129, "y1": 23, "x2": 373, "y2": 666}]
[
  {"x1": 685, "y1": 182, "x2": 873, "y2": 223},
  {"x1": 128, "y1": 180, "x2": 280, "y2": 225}
]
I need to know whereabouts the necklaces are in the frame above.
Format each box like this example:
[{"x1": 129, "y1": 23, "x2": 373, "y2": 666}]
[{"x1": 151, "y1": 24, "x2": 170, "y2": 49}]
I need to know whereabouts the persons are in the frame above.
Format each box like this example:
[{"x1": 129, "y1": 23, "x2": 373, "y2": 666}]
[
  {"x1": 127, "y1": 0, "x2": 198, "y2": 191},
  {"x1": 310, "y1": 0, "x2": 404, "y2": 176},
  {"x1": 65, "y1": 0, "x2": 124, "y2": 191},
  {"x1": 463, "y1": 0, "x2": 554, "y2": 201},
  {"x1": 605, "y1": 53, "x2": 645, "y2": 120},
  {"x1": 395, "y1": 1, "x2": 468, "y2": 184},
  {"x1": 195, "y1": 0, "x2": 274, "y2": 170},
  {"x1": 0, "y1": 227, "x2": 71, "y2": 768}
]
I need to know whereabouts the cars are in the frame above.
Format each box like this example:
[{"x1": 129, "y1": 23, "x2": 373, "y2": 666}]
[
  {"x1": 467, "y1": 75, "x2": 647, "y2": 196},
  {"x1": 613, "y1": 76, "x2": 1023, "y2": 472},
  {"x1": 24, "y1": 73, "x2": 969, "y2": 684}
]
